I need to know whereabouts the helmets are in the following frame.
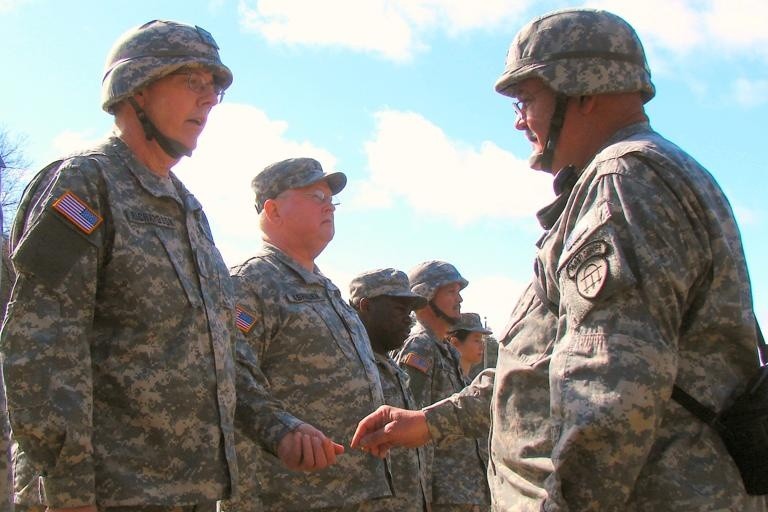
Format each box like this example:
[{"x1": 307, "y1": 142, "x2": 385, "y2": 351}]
[
  {"x1": 407, "y1": 259, "x2": 469, "y2": 303},
  {"x1": 100, "y1": 17, "x2": 232, "y2": 112},
  {"x1": 493, "y1": 10, "x2": 655, "y2": 103}
]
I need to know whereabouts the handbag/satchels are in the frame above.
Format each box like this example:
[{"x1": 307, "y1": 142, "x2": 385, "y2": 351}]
[{"x1": 672, "y1": 316, "x2": 768, "y2": 497}]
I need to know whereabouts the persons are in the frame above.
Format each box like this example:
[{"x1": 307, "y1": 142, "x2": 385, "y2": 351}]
[
  {"x1": 390, "y1": 260, "x2": 490, "y2": 511},
  {"x1": 224, "y1": 156, "x2": 397, "y2": 511},
  {"x1": 0, "y1": 19, "x2": 344, "y2": 510},
  {"x1": 445, "y1": 313, "x2": 492, "y2": 382},
  {"x1": 349, "y1": 7, "x2": 767, "y2": 511},
  {"x1": 349, "y1": 267, "x2": 434, "y2": 511}
]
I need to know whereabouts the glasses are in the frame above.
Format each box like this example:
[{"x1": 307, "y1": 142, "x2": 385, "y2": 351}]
[
  {"x1": 512, "y1": 83, "x2": 551, "y2": 118},
  {"x1": 167, "y1": 68, "x2": 226, "y2": 105},
  {"x1": 312, "y1": 189, "x2": 341, "y2": 207}
]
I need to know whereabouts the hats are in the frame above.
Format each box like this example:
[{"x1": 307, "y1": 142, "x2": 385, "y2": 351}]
[
  {"x1": 251, "y1": 157, "x2": 348, "y2": 214},
  {"x1": 348, "y1": 267, "x2": 428, "y2": 310},
  {"x1": 446, "y1": 312, "x2": 493, "y2": 335}
]
